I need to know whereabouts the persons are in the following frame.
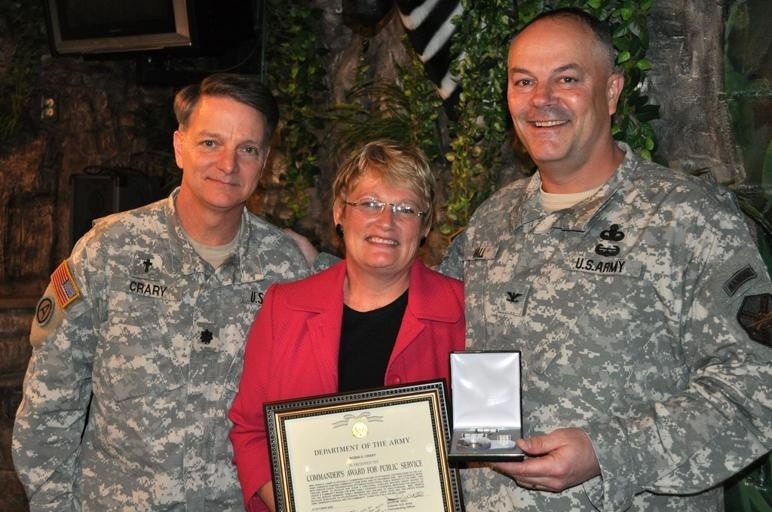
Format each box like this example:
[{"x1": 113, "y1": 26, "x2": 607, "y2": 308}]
[
  {"x1": 226, "y1": 137, "x2": 464, "y2": 512},
  {"x1": 11, "y1": 74, "x2": 314, "y2": 512},
  {"x1": 275, "y1": 7, "x2": 772, "y2": 512}
]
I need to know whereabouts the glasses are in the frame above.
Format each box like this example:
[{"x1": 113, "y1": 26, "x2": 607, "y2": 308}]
[{"x1": 341, "y1": 197, "x2": 426, "y2": 221}]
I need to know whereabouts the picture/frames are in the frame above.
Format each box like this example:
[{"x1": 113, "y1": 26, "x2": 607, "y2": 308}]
[{"x1": 262, "y1": 380, "x2": 466, "y2": 511}]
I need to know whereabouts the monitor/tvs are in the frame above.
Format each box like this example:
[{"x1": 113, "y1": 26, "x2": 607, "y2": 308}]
[{"x1": 47, "y1": 0, "x2": 254, "y2": 55}]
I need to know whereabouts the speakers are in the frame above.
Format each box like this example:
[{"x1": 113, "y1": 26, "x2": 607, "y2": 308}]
[{"x1": 69, "y1": 175, "x2": 120, "y2": 257}]
